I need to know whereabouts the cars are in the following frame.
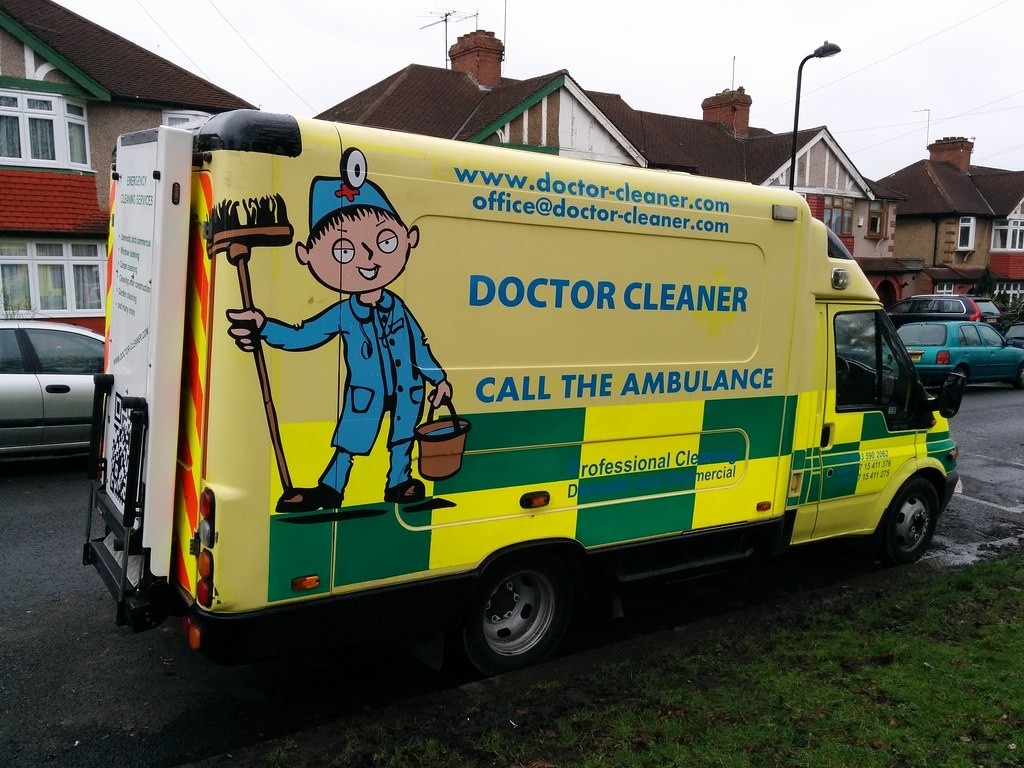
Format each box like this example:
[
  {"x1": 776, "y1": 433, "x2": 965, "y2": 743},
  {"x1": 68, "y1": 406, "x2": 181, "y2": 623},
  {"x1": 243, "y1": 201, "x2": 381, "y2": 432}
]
[
  {"x1": 0, "y1": 319, "x2": 105, "y2": 462},
  {"x1": 886, "y1": 294, "x2": 980, "y2": 328},
  {"x1": 971, "y1": 297, "x2": 1004, "y2": 333},
  {"x1": 880, "y1": 321, "x2": 1024, "y2": 394}
]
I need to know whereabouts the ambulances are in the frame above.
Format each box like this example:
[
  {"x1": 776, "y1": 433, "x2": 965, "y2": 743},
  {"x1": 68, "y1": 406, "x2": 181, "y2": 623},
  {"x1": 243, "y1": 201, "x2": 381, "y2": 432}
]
[{"x1": 81, "y1": 111, "x2": 955, "y2": 676}]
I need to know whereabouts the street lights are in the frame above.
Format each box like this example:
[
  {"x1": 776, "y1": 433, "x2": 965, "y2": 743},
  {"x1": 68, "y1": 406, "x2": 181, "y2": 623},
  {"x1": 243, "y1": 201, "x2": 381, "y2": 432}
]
[{"x1": 789, "y1": 41, "x2": 841, "y2": 190}]
